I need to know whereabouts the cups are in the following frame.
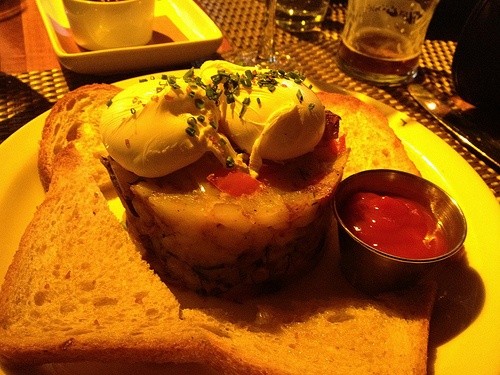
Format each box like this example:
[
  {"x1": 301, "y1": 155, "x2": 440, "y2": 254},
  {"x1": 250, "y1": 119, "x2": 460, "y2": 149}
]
[
  {"x1": 274, "y1": 0, "x2": 328, "y2": 32},
  {"x1": 334, "y1": 169, "x2": 468, "y2": 292},
  {"x1": 62, "y1": 0, "x2": 154, "y2": 51},
  {"x1": 334, "y1": 0, "x2": 439, "y2": 87}
]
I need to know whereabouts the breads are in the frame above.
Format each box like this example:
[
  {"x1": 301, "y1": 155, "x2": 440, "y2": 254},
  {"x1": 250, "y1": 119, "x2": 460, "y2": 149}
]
[
  {"x1": 0, "y1": 141, "x2": 436, "y2": 375},
  {"x1": 39, "y1": 82, "x2": 127, "y2": 192},
  {"x1": 310, "y1": 90, "x2": 421, "y2": 177}
]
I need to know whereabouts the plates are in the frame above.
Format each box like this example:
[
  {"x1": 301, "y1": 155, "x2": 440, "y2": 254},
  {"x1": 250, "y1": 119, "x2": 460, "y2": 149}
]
[
  {"x1": 35, "y1": 0, "x2": 223, "y2": 76},
  {"x1": 1, "y1": 69, "x2": 500, "y2": 375}
]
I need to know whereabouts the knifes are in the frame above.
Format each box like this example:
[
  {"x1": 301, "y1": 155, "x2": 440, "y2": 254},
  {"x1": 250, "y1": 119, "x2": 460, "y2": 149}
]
[{"x1": 407, "y1": 79, "x2": 500, "y2": 169}]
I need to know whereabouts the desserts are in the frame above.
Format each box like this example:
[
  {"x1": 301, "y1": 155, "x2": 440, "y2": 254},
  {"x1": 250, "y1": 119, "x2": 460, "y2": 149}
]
[{"x1": 97, "y1": 57, "x2": 348, "y2": 295}]
